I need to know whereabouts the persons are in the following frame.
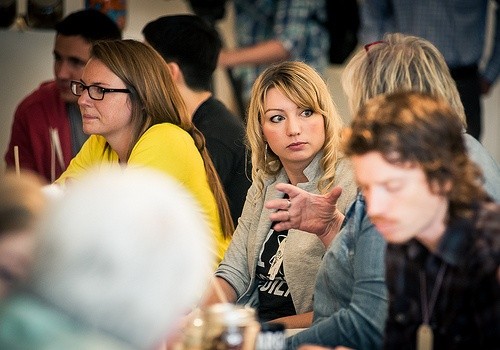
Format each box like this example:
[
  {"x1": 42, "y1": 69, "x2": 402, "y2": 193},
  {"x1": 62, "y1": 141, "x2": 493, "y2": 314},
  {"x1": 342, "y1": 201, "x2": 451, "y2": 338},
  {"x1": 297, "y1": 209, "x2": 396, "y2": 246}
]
[
  {"x1": 207, "y1": 64, "x2": 359, "y2": 331},
  {"x1": 5, "y1": 9, "x2": 122, "y2": 187},
  {"x1": 39, "y1": 40, "x2": 234, "y2": 270},
  {"x1": 141, "y1": 14, "x2": 258, "y2": 234},
  {"x1": 215, "y1": 0, "x2": 330, "y2": 115},
  {"x1": 0, "y1": 168, "x2": 46, "y2": 301},
  {"x1": 341, "y1": 89, "x2": 500, "y2": 350},
  {"x1": 359, "y1": 0, "x2": 500, "y2": 145},
  {"x1": 0, "y1": 167, "x2": 215, "y2": 350},
  {"x1": 266, "y1": 32, "x2": 500, "y2": 350}
]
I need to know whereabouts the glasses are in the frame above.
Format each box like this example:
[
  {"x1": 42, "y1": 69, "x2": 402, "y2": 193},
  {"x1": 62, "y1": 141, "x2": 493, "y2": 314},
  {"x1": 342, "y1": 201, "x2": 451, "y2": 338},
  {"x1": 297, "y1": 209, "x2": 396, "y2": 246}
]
[
  {"x1": 364, "y1": 41, "x2": 387, "y2": 64},
  {"x1": 71, "y1": 80, "x2": 131, "y2": 101}
]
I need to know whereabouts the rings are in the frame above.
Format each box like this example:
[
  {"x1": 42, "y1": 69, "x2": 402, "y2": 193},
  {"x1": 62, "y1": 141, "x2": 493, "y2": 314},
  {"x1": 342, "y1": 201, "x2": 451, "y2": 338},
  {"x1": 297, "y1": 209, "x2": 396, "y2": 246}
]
[
  {"x1": 286, "y1": 211, "x2": 290, "y2": 221},
  {"x1": 287, "y1": 199, "x2": 291, "y2": 209}
]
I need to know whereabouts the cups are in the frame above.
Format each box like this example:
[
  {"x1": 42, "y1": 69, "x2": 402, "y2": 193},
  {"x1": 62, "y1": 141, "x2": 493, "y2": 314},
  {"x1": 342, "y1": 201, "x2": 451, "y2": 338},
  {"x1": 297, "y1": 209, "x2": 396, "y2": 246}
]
[{"x1": 161, "y1": 302, "x2": 260, "y2": 349}]
